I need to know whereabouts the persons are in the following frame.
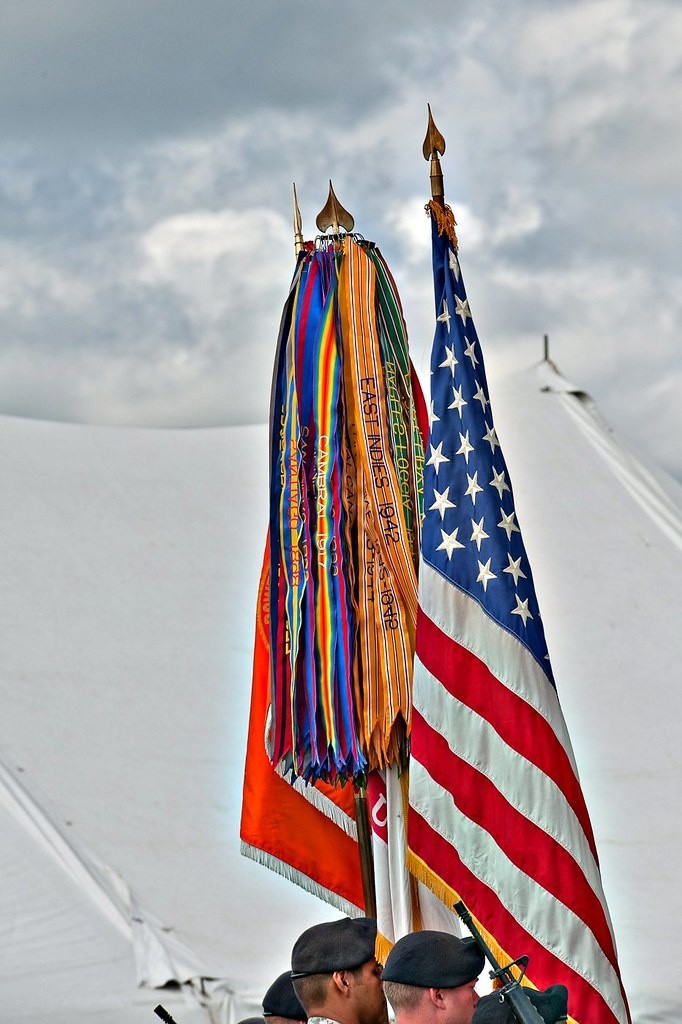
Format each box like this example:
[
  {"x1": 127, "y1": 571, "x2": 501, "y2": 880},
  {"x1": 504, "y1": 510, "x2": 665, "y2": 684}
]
[
  {"x1": 289, "y1": 916, "x2": 389, "y2": 1024},
  {"x1": 469, "y1": 981, "x2": 567, "y2": 1024},
  {"x1": 380, "y1": 930, "x2": 486, "y2": 1024},
  {"x1": 263, "y1": 970, "x2": 308, "y2": 1024}
]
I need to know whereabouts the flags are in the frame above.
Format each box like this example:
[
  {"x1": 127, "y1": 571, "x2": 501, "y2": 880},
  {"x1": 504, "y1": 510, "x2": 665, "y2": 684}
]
[
  {"x1": 239, "y1": 225, "x2": 468, "y2": 963},
  {"x1": 398, "y1": 207, "x2": 636, "y2": 1024}
]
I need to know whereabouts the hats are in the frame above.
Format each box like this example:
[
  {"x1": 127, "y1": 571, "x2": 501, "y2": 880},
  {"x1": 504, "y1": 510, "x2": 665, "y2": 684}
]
[
  {"x1": 472, "y1": 985, "x2": 568, "y2": 1024},
  {"x1": 292, "y1": 917, "x2": 378, "y2": 970},
  {"x1": 263, "y1": 971, "x2": 310, "y2": 1021},
  {"x1": 380, "y1": 931, "x2": 484, "y2": 989}
]
